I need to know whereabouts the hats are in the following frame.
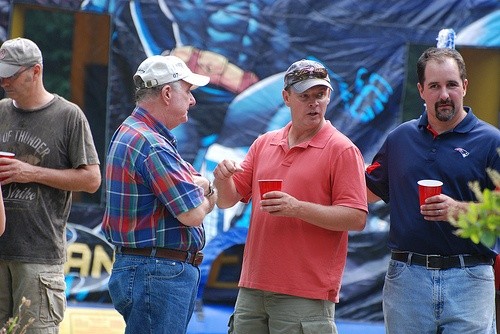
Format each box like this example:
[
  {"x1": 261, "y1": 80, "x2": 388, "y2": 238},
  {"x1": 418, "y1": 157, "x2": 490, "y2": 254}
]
[
  {"x1": 133, "y1": 55, "x2": 211, "y2": 91},
  {"x1": 283, "y1": 58, "x2": 335, "y2": 95},
  {"x1": 0, "y1": 38, "x2": 44, "y2": 78}
]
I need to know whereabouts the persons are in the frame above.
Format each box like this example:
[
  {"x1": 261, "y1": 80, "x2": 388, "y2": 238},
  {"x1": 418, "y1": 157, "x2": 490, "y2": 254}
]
[
  {"x1": 214, "y1": 60, "x2": 368, "y2": 334},
  {"x1": 102, "y1": 56, "x2": 217, "y2": 334},
  {"x1": 365, "y1": 49, "x2": 500, "y2": 334},
  {"x1": 0, "y1": 37, "x2": 102, "y2": 334}
]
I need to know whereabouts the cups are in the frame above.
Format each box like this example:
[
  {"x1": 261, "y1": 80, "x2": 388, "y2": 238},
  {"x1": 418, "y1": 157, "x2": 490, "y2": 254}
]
[
  {"x1": 259, "y1": 179, "x2": 282, "y2": 207},
  {"x1": 417, "y1": 179, "x2": 443, "y2": 214},
  {"x1": 0, "y1": 151, "x2": 15, "y2": 182}
]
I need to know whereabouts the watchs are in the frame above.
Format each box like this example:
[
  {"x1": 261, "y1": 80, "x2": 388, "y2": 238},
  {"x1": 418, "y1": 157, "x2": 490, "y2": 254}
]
[{"x1": 206, "y1": 180, "x2": 214, "y2": 197}]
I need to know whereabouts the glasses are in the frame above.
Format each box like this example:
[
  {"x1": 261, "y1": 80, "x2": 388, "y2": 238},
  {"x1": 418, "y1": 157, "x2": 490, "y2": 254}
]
[
  {"x1": 285, "y1": 68, "x2": 329, "y2": 91},
  {"x1": 0, "y1": 66, "x2": 31, "y2": 83}
]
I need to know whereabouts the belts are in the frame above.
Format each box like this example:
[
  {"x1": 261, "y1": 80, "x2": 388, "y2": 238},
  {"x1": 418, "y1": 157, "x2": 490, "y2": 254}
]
[
  {"x1": 116, "y1": 247, "x2": 204, "y2": 267},
  {"x1": 390, "y1": 252, "x2": 495, "y2": 270}
]
